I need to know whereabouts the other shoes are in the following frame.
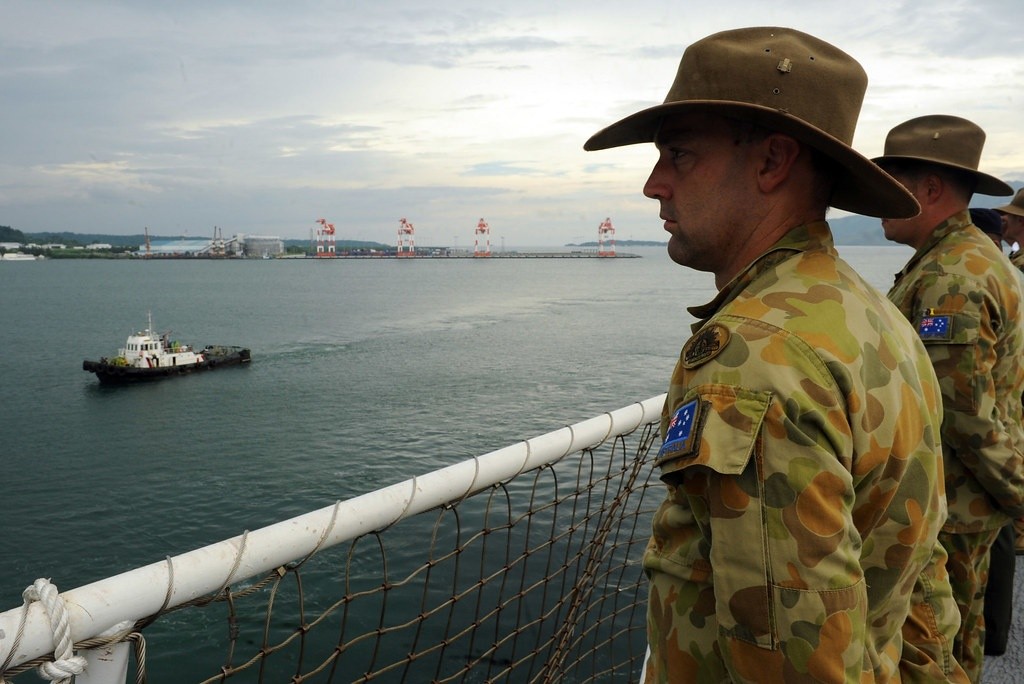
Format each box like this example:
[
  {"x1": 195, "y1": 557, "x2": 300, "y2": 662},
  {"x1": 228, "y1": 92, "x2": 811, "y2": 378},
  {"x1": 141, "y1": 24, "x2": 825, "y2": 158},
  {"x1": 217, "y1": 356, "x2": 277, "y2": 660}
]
[
  {"x1": 1013, "y1": 520, "x2": 1024, "y2": 555},
  {"x1": 984, "y1": 649, "x2": 1004, "y2": 656}
]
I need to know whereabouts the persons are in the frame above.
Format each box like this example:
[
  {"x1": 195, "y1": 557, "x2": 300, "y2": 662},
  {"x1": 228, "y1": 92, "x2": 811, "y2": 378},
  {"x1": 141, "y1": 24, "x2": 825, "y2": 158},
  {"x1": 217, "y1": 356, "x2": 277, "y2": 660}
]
[
  {"x1": 868, "y1": 114, "x2": 1024, "y2": 684},
  {"x1": 966, "y1": 187, "x2": 1024, "y2": 268},
  {"x1": 584, "y1": 25, "x2": 973, "y2": 684}
]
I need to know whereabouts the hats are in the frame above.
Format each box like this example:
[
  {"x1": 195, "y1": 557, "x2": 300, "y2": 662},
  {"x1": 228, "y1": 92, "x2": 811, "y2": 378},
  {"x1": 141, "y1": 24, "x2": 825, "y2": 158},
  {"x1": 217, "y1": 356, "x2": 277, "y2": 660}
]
[
  {"x1": 869, "y1": 115, "x2": 1014, "y2": 196},
  {"x1": 583, "y1": 26, "x2": 922, "y2": 220},
  {"x1": 969, "y1": 208, "x2": 1002, "y2": 236},
  {"x1": 997, "y1": 187, "x2": 1024, "y2": 217}
]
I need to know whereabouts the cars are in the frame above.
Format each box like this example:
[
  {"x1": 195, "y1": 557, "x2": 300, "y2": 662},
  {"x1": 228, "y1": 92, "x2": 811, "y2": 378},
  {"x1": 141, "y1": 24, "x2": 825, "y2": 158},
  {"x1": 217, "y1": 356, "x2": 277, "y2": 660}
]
[{"x1": 82, "y1": 310, "x2": 252, "y2": 381}]
